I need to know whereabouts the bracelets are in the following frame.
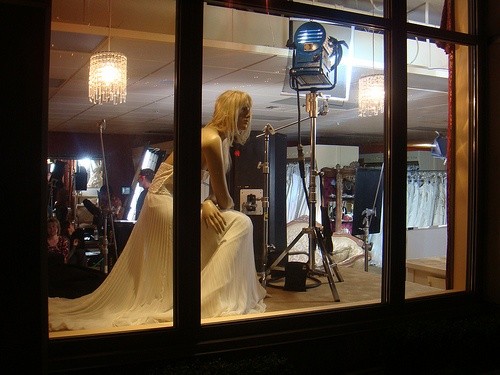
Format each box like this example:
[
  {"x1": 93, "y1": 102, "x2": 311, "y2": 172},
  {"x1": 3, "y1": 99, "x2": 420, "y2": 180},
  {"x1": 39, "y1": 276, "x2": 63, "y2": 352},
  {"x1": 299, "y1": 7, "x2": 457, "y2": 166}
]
[{"x1": 73, "y1": 245, "x2": 77, "y2": 247}]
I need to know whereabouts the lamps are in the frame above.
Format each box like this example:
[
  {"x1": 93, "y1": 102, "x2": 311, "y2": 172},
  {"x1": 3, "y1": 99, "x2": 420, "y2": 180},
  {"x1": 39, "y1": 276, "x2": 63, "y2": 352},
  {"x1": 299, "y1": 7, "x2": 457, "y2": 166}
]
[
  {"x1": 87, "y1": 0, "x2": 128, "y2": 105},
  {"x1": 358, "y1": 5, "x2": 387, "y2": 118}
]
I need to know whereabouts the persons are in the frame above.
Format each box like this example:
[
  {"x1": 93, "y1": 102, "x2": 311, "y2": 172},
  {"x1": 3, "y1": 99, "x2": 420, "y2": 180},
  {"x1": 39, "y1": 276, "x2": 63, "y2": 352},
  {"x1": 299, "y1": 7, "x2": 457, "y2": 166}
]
[
  {"x1": 49, "y1": 169, "x2": 155, "y2": 264},
  {"x1": 48, "y1": 90, "x2": 267, "y2": 331}
]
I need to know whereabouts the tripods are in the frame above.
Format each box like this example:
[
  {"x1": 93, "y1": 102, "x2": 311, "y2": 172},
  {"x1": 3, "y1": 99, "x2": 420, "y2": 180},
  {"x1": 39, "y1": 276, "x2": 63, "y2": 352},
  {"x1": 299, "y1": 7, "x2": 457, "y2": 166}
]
[{"x1": 259, "y1": 89, "x2": 345, "y2": 303}]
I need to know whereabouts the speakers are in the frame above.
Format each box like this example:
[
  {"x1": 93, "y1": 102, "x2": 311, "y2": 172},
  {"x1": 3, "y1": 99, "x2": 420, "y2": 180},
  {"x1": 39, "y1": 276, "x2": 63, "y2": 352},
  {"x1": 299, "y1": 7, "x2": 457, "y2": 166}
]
[{"x1": 231, "y1": 129, "x2": 288, "y2": 282}]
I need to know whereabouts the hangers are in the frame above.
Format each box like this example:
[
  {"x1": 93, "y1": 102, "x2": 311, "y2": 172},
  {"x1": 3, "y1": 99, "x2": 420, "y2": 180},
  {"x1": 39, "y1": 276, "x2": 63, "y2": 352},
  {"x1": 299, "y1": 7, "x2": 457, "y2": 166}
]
[{"x1": 410, "y1": 169, "x2": 443, "y2": 178}]
